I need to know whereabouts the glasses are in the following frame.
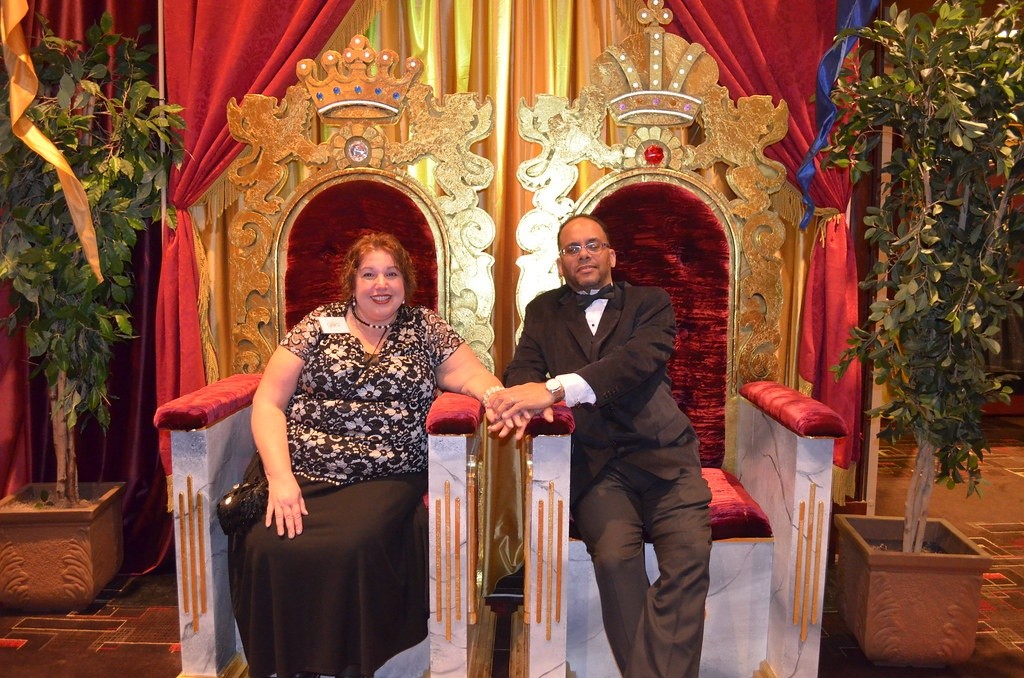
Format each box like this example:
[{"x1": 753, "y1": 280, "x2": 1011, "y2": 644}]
[{"x1": 558, "y1": 242, "x2": 611, "y2": 256}]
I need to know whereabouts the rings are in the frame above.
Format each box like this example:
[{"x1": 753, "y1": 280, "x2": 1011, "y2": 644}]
[{"x1": 511, "y1": 397, "x2": 515, "y2": 405}]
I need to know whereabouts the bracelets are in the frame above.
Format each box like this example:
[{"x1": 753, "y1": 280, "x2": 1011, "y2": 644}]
[{"x1": 482, "y1": 387, "x2": 507, "y2": 407}]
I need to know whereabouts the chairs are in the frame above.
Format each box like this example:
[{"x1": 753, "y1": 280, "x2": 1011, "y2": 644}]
[
  {"x1": 154, "y1": 31, "x2": 496, "y2": 678},
  {"x1": 511, "y1": 0, "x2": 848, "y2": 678}
]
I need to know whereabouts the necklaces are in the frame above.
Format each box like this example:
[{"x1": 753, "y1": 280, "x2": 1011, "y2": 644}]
[{"x1": 352, "y1": 305, "x2": 393, "y2": 328}]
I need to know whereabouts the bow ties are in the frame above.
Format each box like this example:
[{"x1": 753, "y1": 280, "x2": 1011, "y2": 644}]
[{"x1": 575, "y1": 283, "x2": 614, "y2": 311}]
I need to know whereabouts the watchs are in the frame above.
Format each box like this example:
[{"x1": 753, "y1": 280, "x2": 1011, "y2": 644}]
[{"x1": 546, "y1": 379, "x2": 562, "y2": 403}]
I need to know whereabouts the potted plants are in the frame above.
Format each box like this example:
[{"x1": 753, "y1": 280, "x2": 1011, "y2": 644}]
[
  {"x1": 0, "y1": 10, "x2": 193, "y2": 616},
  {"x1": 811, "y1": 0, "x2": 1023, "y2": 670}
]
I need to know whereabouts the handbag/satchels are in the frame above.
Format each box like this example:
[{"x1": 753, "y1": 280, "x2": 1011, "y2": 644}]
[{"x1": 217, "y1": 476, "x2": 268, "y2": 534}]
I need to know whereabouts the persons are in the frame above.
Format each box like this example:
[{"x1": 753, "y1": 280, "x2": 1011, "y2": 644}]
[
  {"x1": 487, "y1": 213, "x2": 712, "y2": 678},
  {"x1": 228, "y1": 234, "x2": 530, "y2": 678}
]
